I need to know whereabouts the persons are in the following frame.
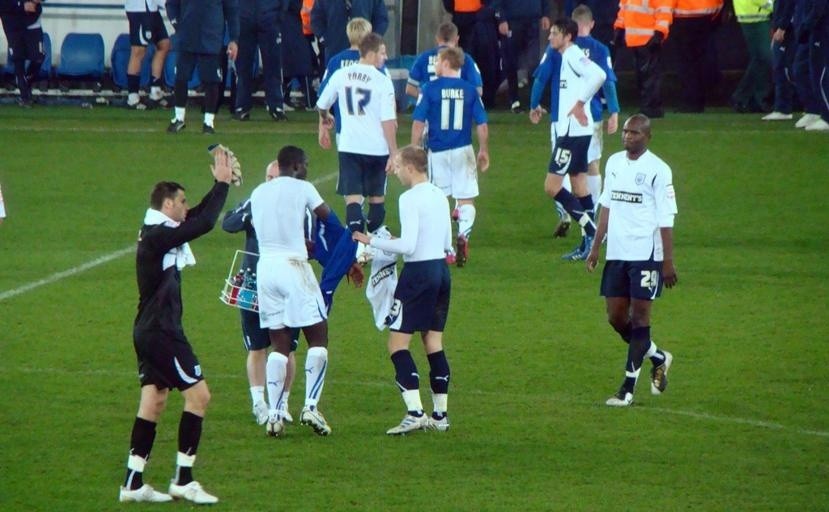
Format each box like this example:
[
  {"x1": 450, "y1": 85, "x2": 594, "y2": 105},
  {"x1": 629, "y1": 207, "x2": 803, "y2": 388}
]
[
  {"x1": 249, "y1": 143, "x2": 365, "y2": 440},
  {"x1": 221, "y1": 157, "x2": 319, "y2": 428},
  {"x1": 544, "y1": 17, "x2": 609, "y2": 264},
  {"x1": 584, "y1": 112, "x2": 682, "y2": 409},
  {"x1": 117, "y1": 145, "x2": 234, "y2": 506},
  {"x1": 441, "y1": 1, "x2": 828, "y2": 132},
  {"x1": 408, "y1": 46, "x2": 492, "y2": 269},
  {"x1": 350, "y1": 144, "x2": 455, "y2": 435},
  {"x1": 527, "y1": 4, "x2": 622, "y2": 239},
  {"x1": 1, "y1": 1, "x2": 46, "y2": 109},
  {"x1": 405, "y1": 21, "x2": 483, "y2": 101},
  {"x1": 124, "y1": 1, "x2": 396, "y2": 136},
  {"x1": 316, "y1": 33, "x2": 400, "y2": 266}
]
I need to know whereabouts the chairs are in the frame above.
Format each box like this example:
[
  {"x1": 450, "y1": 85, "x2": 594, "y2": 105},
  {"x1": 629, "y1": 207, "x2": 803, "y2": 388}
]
[
  {"x1": 111, "y1": 34, "x2": 155, "y2": 94},
  {"x1": 4, "y1": 32, "x2": 51, "y2": 91},
  {"x1": 55, "y1": 33, "x2": 104, "y2": 92},
  {"x1": 164, "y1": 34, "x2": 200, "y2": 94}
]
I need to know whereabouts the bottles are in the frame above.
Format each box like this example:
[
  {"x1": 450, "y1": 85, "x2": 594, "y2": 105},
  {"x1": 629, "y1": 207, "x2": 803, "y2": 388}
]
[{"x1": 224, "y1": 267, "x2": 258, "y2": 311}]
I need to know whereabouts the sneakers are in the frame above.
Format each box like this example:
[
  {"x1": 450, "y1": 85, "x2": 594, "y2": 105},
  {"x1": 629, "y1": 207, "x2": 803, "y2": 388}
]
[
  {"x1": 251, "y1": 401, "x2": 294, "y2": 426},
  {"x1": 298, "y1": 405, "x2": 331, "y2": 436},
  {"x1": 553, "y1": 223, "x2": 598, "y2": 260},
  {"x1": 120, "y1": 484, "x2": 174, "y2": 504},
  {"x1": 426, "y1": 412, "x2": 450, "y2": 430},
  {"x1": 651, "y1": 349, "x2": 672, "y2": 394},
  {"x1": 452, "y1": 208, "x2": 471, "y2": 268},
  {"x1": 353, "y1": 241, "x2": 376, "y2": 267},
  {"x1": 264, "y1": 410, "x2": 286, "y2": 438},
  {"x1": 605, "y1": 385, "x2": 634, "y2": 407},
  {"x1": 510, "y1": 101, "x2": 526, "y2": 116},
  {"x1": 122, "y1": 95, "x2": 318, "y2": 136},
  {"x1": 386, "y1": 411, "x2": 428, "y2": 433},
  {"x1": 761, "y1": 108, "x2": 827, "y2": 132},
  {"x1": 168, "y1": 479, "x2": 220, "y2": 504}
]
